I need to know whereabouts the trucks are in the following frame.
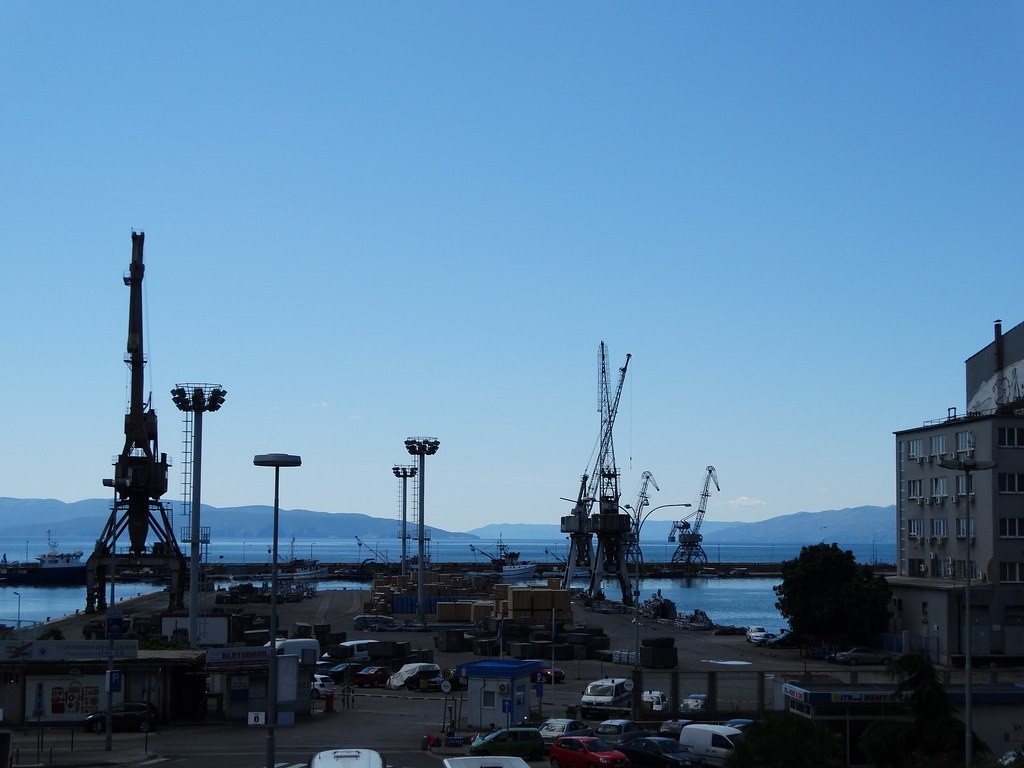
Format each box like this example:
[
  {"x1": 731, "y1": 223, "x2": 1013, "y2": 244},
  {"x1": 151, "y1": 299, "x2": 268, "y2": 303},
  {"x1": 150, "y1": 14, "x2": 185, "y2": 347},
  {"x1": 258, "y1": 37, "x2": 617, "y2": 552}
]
[
  {"x1": 442, "y1": 755, "x2": 536, "y2": 768},
  {"x1": 310, "y1": 746, "x2": 387, "y2": 768},
  {"x1": 339, "y1": 640, "x2": 379, "y2": 660},
  {"x1": 680, "y1": 724, "x2": 745, "y2": 768},
  {"x1": 264, "y1": 637, "x2": 320, "y2": 667}
]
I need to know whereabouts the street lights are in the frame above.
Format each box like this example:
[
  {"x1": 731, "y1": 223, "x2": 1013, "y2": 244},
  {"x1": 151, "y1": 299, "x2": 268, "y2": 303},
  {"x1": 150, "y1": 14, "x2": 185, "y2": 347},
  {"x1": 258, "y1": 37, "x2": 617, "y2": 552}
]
[
  {"x1": 391, "y1": 462, "x2": 419, "y2": 575},
  {"x1": 936, "y1": 454, "x2": 999, "y2": 768},
  {"x1": 171, "y1": 379, "x2": 227, "y2": 648},
  {"x1": 250, "y1": 450, "x2": 303, "y2": 768},
  {"x1": 406, "y1": 434, "x2": 441, "y2": 620},
  {"x1": 583, "y1": 495, "x2": 693, "y2": 670},
  {"x1": 14, "y1": 591, "x2": 22, "y2": 629},
  {"x1": 103, "y1": 478, "x2": 131, "y2": 750}
]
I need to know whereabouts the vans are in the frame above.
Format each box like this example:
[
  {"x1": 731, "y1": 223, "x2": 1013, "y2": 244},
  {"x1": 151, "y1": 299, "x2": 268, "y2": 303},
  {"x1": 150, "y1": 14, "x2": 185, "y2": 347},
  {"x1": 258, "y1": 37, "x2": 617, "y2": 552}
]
[{"x1": 579, "y1": 677, "x2": 632, "y2": 719}]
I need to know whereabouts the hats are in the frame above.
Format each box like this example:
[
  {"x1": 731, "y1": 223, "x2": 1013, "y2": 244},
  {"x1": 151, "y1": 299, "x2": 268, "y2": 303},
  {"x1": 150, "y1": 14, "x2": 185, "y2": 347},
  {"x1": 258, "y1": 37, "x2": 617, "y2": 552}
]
[{"x1": 489, "y1": 723, "x2": 496, "y2": 729}]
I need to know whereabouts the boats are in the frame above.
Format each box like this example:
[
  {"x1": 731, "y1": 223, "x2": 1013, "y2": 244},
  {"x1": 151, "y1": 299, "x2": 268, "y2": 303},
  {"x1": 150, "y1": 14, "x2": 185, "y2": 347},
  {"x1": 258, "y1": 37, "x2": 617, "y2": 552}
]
[
  {"x1": 463, "y1": 534, "x2": 539, "y2": 581},
  {"x1": 251, "y1": 542, "x2": 330, "y2": 582},
  {"x1": 541, "y1": 547, "x2": 591, "y2": 578}
]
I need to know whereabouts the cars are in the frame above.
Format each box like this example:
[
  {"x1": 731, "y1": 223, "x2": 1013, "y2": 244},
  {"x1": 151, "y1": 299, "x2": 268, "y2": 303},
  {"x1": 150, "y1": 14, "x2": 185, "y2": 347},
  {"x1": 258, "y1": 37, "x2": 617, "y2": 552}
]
[
  {"x1": 353, "y1": 666, "x2": 393, "y2": 687},
  {"x1": 723, "y1": 719, "x2": 755, "y2": 731},
  {"x1": 746, "y1": 625, "x2": 803, "y2": 649},
  {"x1": 311, "y1": 661, "x2": 334, "y2": 675},
  {"x1": 679, "y1": 694, "x2": 706, "y2": 710},
  {"x1": 817, "y1": 646, "x2": 891, "y2": 667},
  {"x1": 538, "y1": 718, "x2": 594, "y2": 750},
  {"x1": 309, "y1": 674, "x2": 335, "y2": 699},
  {"x1": 520, "y1": 659, "x2": 565, "y2": 683},
  {"x1": 327, "y1": 663, "x2": 362, "y2": 684},
  {"x1": 469, "y1": 728, "x2": 545, "y2": 760},
  {"x1": 642, "y1": 690, "x2": 667, "y2": 712},
  {"x1": 428, "y1": 668, "x2": 469, "y2": 693},
  {"x1": 597, "y1": 719, "x2": 644, "y2": 744},
  {"x1": 614, "y1": 737, "x2": 708, "y2": 768},
  {"x1": 548, "y1": 734, "x2": 630, "y2": 768},
  {"x1": 82, "y1": 702, "x2": 155, "y2": 734}
]
